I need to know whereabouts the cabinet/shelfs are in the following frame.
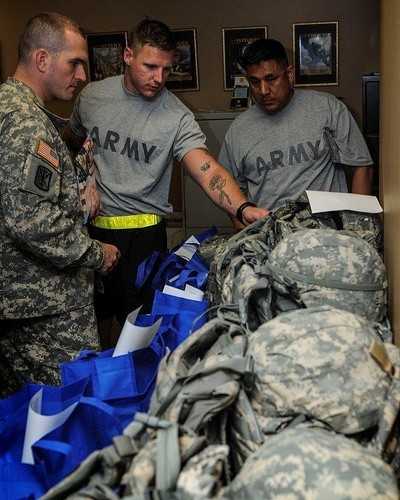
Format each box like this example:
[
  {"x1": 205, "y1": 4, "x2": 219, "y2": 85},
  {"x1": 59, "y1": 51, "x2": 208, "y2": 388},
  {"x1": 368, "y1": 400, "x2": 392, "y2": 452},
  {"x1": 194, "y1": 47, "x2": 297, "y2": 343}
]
[{"x1": 181, "y1": 112, "x2": 242, "y2": 241}]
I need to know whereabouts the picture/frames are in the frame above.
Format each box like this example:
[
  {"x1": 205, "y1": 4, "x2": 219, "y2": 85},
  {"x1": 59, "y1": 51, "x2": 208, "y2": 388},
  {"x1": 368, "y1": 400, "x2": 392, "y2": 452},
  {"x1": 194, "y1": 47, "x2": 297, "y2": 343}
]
[
  {"x1": 222, "y1": 25, "x2": 268, "y2": 91},
  {"x1": 84, "y1": 31, "x2": 128, "y2": 82},
  {"x1": 164, "y1": 28, "x2": 200, "y2": 93},
  {"x1": 292, "y1": 21, "x2": 339, "y2": 87}
]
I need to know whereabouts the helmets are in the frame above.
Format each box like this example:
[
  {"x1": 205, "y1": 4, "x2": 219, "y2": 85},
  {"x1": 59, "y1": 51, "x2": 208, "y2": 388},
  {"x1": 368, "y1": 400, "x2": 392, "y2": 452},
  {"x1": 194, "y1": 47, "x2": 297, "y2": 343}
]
[
  {"x1": 265, "y1": 228, "x2": 388, "y2": 326},
  {"x1": 244, "y1": 305, "x2": 393, "y2": 434},
  {"x1": 230, "y1": 427, "x2": 399, "y2": 500}
]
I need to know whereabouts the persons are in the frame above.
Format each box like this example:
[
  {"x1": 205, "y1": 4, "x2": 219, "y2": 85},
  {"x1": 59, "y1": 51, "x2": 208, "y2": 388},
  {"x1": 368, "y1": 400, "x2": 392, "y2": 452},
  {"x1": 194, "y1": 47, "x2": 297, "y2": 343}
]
[
  {"x1": 62, "y1": 15, "x2": 271, "y2": 351},
  {"x1": 217, "y1": 39, "x2": 372, "y2": 231},
  {"x1": 0, "y1": 12, "x2": 120, "y2": 400}
]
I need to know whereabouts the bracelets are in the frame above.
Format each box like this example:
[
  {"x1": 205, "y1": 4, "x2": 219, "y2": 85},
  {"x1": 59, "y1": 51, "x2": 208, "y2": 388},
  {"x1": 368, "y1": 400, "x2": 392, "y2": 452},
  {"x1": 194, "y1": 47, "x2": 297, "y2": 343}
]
[{"x1": 236, "y1": 201, "x2": 257, "y2": 225}]
